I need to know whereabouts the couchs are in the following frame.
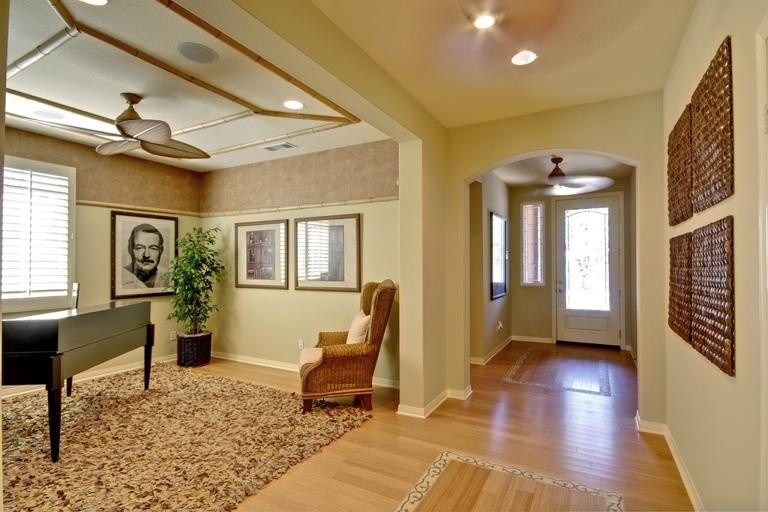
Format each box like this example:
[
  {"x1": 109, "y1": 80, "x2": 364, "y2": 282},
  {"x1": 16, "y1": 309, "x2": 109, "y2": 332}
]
[{"x1": 298, "y1": 277, "x2": 396, "y2": 412}]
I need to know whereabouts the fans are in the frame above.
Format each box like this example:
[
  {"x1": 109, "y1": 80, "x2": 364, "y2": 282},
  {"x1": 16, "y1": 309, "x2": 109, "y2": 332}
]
[{"x1": 95, "y1": 92, "x2": 211, "y2": 159}]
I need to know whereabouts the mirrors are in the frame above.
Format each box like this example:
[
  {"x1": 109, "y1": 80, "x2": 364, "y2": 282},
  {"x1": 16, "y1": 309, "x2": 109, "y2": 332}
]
[
  {"x1": 293, "y1": 213, "x2": 361, "y2": 293},
  {"x1": 489, "y1": 211, "x2": 508, "y2": 300}
]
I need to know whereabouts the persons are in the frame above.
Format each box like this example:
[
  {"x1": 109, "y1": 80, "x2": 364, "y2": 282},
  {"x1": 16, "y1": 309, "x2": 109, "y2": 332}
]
[{"x1": 120, "y1": 224, "x2": 170, "y2": 290}]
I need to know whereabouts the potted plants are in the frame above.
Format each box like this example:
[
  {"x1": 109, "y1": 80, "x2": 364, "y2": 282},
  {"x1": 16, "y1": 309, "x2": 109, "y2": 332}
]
[{"x1": 160, "y1": 226, "x2": 226, "y2": 368}]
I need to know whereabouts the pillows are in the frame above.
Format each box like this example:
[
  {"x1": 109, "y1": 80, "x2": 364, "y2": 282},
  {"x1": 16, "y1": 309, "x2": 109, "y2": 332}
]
[{"x1": 347, "y1": 311, "x2": 370, "y2": 345}]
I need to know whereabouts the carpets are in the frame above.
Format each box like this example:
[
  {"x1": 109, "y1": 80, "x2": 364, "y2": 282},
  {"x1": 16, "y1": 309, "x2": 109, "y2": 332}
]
[{"x1": 0, "y1": 361, "x2": 373, "y2": 512}]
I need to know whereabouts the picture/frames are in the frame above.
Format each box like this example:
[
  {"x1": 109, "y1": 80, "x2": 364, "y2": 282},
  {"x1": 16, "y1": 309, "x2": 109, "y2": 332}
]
[
  {"x1": 234, "y1": 218, "x2": 289, "y2": 290},
  {"x1": 110, "y1": 210, "x2": 179, "y2": 300}
]
